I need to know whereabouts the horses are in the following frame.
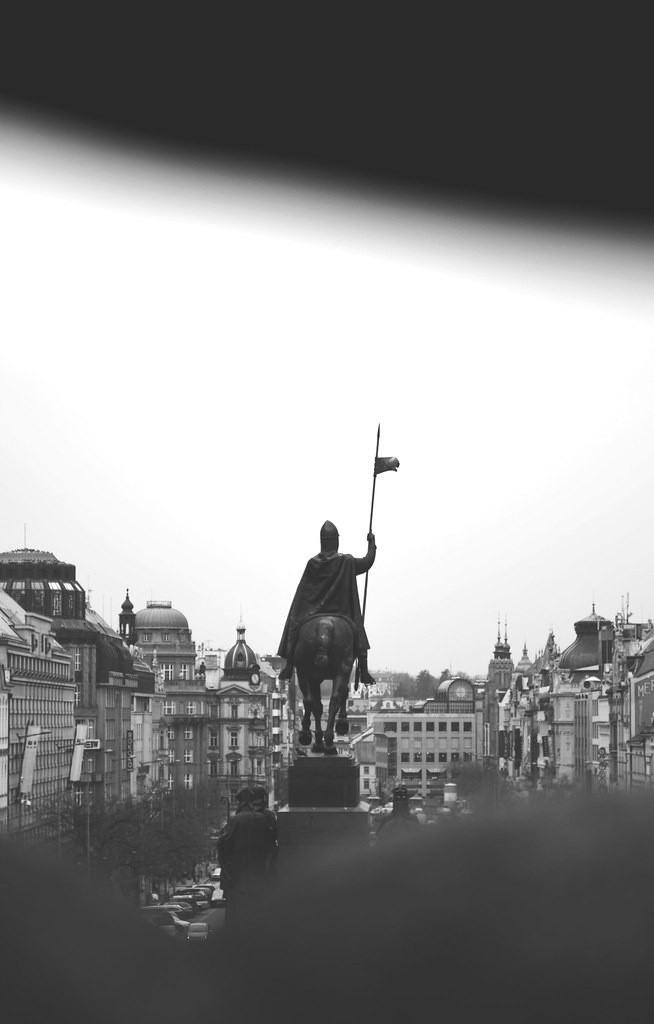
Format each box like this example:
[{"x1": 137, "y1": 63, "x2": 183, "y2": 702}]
[{"x1": 292, "y1": 616, "x2": 355, "y2": 757}]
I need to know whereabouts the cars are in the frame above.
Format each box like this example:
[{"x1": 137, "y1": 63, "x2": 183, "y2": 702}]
[
  {"x1": 186, "y1": 922, "x2": 210, "y2": 945},
  {"x1": 146, "y1": 868, "x2": 226, "y2": 931}
]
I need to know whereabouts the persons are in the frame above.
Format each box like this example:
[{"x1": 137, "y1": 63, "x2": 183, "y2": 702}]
[
  {"x1": 276, "y1": 520, "x2": 377, "y2": 684},
  {"x1": 217, "y1": 785, "x2": 277, "y2": 932},
  {"x1": 375, "y1": 787, "x2": 420, "y2": 842}
]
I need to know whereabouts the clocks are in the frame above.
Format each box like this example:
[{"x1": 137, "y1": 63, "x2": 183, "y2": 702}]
[{"x1": 250, "y1": 673, "x2": 260, "y2": 685}]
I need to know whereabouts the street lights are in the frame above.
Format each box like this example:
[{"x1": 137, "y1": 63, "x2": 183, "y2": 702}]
[
  {"x1": 56, "y1": 741, "x2": 91, "y2": 862},
  {"x1": 139, "y1": 759, "x2": 162, "y2": 895},
  {"x1": 15, "y1": 731, "x2": 51, "y2": 832},
  {"x1": 160, "y1": 759, "x2": 233, "y2": 899},
  {"x1": 111, "y1": 754, "x2": 136, "y2": 825},
  {"x1": 88, "y1": 749, "x2": 114, "y2": 877}
]
